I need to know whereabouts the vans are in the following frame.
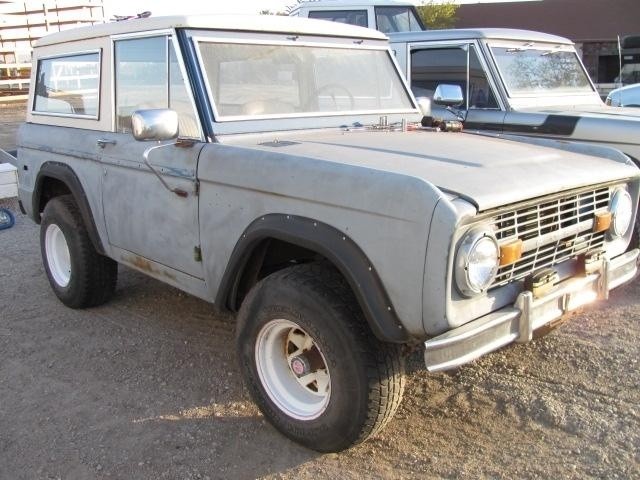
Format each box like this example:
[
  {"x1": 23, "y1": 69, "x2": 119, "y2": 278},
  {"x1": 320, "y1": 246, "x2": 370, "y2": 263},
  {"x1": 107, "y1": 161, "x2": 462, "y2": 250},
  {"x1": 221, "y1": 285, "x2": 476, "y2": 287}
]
[{"x1": 289, "y1": 2, "x2": 428, "y2": 35}]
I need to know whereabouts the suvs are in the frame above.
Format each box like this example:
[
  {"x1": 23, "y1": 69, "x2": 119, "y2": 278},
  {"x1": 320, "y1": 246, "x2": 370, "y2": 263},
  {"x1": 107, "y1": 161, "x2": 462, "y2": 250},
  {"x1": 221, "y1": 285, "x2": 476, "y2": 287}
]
[
  {"x1": 383, "y1": 27, "x2": 640, "y2": 170},
  {"x1": 13, "y1": 15, "x2": 639, "y2": 455}
]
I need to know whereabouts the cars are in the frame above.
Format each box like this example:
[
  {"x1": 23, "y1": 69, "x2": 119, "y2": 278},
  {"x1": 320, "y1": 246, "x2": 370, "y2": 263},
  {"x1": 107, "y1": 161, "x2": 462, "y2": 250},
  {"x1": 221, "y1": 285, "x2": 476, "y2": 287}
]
[{"x1": 604, "y1": 81, "x2": 640, "y2": 108}]
